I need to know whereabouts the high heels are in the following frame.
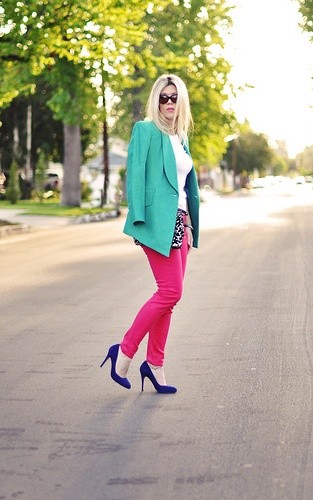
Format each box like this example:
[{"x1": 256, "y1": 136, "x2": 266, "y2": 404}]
[
  {"x1": 100, "y1": 344, "x2": 131, "y2": 389},
  {"x1": 140, "y1": 361, "x2": 177, "y2": 394}
]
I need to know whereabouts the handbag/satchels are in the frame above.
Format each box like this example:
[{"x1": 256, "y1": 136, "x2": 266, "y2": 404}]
[{"x1": 134, "y1": 210, "x2": 188, "y2": 248}]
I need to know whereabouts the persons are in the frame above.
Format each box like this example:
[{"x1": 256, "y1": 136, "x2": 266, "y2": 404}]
[{"x1": 99, "y1": 74, "x2": 200, "y2": 393}]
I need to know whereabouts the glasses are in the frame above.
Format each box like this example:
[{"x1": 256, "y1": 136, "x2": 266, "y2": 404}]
[{"x1": 159, "y1": 94, "x2": 177, "y2": 104}]
